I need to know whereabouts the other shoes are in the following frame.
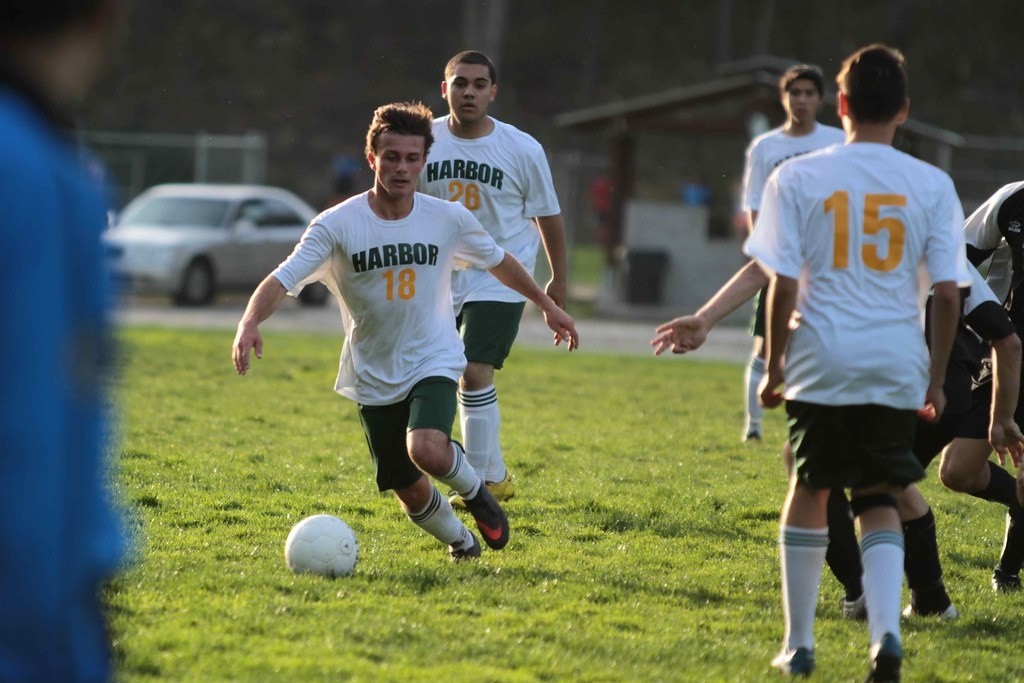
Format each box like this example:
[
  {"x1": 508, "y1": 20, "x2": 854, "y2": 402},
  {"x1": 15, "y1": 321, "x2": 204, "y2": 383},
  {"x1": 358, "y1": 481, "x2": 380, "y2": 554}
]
[{"x1": 744, "y1": 432, "x2": 760, "y2": 441}]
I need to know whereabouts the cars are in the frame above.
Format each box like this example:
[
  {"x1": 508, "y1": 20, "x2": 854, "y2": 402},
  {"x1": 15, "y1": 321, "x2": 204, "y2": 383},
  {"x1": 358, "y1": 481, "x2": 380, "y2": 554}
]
[{"x1": 95, "y1": 181, "x2": 330, "y2": 306}]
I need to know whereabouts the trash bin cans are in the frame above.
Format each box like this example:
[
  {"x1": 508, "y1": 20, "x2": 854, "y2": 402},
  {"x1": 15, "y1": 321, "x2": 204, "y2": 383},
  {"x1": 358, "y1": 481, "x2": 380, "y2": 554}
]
[{"x1": 616, "y1": 250, "x2": 671, "y2": 305}]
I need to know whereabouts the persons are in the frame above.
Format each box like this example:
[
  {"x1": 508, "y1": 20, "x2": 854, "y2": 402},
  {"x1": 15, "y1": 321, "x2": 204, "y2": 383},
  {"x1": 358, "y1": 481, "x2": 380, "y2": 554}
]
[
  {"x1": 421, "y1": 51, "x2": 572, "y2": 513},
  {"x1": 232, "y1": 103, "x2": 580, "y2": 559},
  {"x1": 1, "y1": 0, "x2": 147, "y2": 683},
  {"x1": 597, "y1": 44, "x2": 1024, "y2": 683}
]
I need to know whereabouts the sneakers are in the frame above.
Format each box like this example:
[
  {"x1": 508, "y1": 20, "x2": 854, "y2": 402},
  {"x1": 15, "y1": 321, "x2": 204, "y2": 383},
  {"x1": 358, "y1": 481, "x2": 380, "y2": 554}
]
[
  {"x1": 774, "y1": 645, "x2": 816, "y2": 677},
  {"x1": 447, "y1": 470, "x2": 515, "y2": 509},
  {"x1": 462, "y1": 476, "x2": 510, "y2": 550},
  {"x1": 902, "y1": 603, "x2": 959, "y2": 620},
  {"x1": 867, "y1": 632, "x2": 902, "y2": 683},
  {"x1": 991, "y1": 567, "x2": 1021, "y2": 594},
  {"x1": 842, "y1": 589, "x2": 867, "y2": 622},
  {"x1": 448, "y1": 530, "x2": 481, "y2": 559}
]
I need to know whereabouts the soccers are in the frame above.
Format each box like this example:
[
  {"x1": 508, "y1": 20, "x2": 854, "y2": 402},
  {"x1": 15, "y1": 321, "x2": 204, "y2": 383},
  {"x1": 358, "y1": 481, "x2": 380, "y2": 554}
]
[{"x1": 284, "y1": 513, "x2": 359, "y2": 578}]
